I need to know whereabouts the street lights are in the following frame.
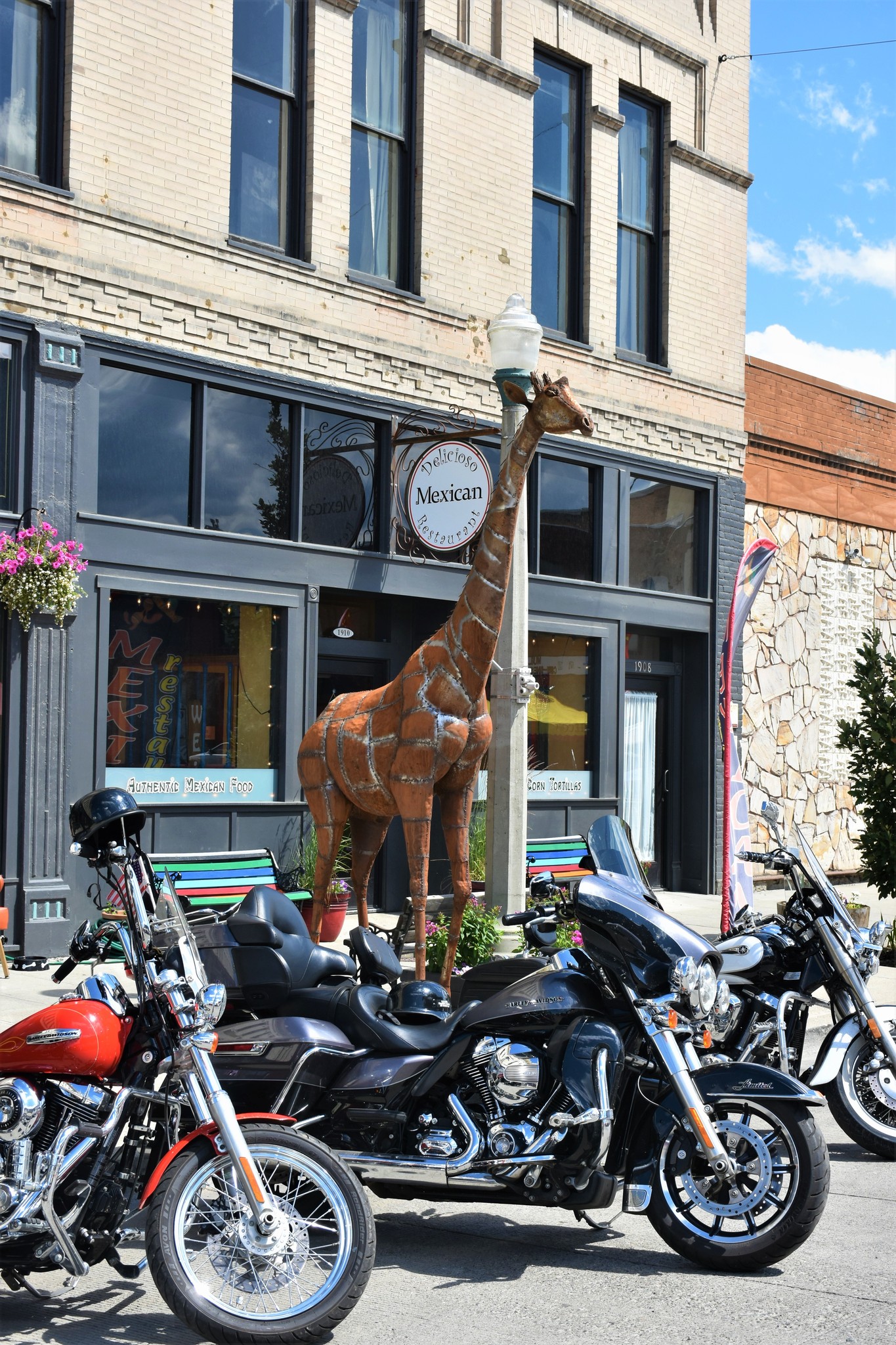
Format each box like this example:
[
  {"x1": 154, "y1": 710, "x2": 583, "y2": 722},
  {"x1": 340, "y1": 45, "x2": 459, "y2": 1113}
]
[{"x1": 485, "y1": 293, "x2": 546, "y2": 955}]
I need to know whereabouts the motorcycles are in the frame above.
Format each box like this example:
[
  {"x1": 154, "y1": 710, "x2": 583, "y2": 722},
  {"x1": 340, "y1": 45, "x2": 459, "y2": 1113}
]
[
  {"x1": 153, "y1": 814, "x2": 835, "y2": 1274},
  {"x1": 455, "y1": 813, "x2": 896, "y2": 1160},
  {"x1": 0, "y1": 789, "x2": 381, "y2": 1345}
]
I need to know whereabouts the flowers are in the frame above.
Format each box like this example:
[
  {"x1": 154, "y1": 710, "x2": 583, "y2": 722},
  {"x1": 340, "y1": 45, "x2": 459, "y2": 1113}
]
[
  {"x1": 424, "y1": 894, "x2": 504, "y2": 975},
  {"x1": 105, "y1": 901, "x2": 127, "y2": 915},
  {"x1": 284, "y1": 812, "x2": 356, "y2": 913},
  {"x1": 511, "y1": 891, "x2": 583, "y2": 957},
  {"x1": 0, "y1": 523, "x2": 88, "y2": 631}
]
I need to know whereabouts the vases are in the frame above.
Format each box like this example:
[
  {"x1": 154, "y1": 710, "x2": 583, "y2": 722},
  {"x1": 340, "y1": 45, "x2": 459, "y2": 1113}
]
[
  {"x1": 102, "y1": 912, "x2": 128, "y2": 920},
  {"x1": 297, "y1": 891, "x2": 353, "y2": 942}
]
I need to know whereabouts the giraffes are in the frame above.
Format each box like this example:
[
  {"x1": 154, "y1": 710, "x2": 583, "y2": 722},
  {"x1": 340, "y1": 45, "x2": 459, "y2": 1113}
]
[{"x1": 289, "y1": 364, "x2": 599, "y2": 996}]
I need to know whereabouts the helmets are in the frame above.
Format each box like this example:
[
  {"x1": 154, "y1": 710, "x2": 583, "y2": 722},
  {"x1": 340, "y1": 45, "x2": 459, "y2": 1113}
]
[
  {"x1": 72, "y1": 788, "x2": 149, "y2": 853},
  {"x1": 374, "y1": 979, "x2": 452, "y2": 1025}
]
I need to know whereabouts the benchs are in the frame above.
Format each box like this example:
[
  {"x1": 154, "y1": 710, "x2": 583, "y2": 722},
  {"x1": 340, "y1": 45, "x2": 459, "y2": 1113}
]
[
  {"x1": 342, "y1": 891, "x2": 485, "y2": 981},
  {"x1": 526, "y1": 834, "x2": 596, "y2": 897},
  {"x1": 146, "y1": 847, "x2": 314, "y2": 915}
]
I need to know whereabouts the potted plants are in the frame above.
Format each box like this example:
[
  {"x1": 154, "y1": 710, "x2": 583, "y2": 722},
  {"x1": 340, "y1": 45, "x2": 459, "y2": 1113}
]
[{"x1": 431, "y1": 733, "x2": 561, "y2": 892}]
